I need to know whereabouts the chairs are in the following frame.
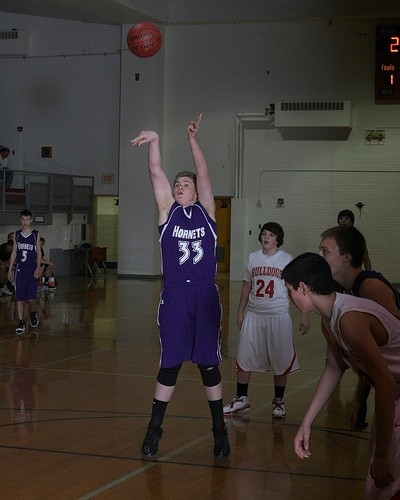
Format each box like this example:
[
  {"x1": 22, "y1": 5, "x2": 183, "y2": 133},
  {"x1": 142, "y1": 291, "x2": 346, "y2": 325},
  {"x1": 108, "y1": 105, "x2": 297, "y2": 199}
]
[{"x1": 87, "y1": 247, "x2": 108, "y2": 274}]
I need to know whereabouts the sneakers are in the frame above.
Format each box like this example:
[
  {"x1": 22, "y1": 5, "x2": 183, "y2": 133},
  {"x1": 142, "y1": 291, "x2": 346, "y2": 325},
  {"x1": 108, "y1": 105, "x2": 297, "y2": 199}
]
[
  {"x1": 222, "y1": 394, "x2": 253, "y2": 415},
  {"x1": 16, "y1": 320, "x2": 25, "y2": 332},
  {"x1": 271, "y1": 399, "x2": 286, "y2": 417},
  {"x1": 211, "y1": 426, "x2": 231, "y2": 460},
  {"x1": 140, "y1": 422, "x2": 165, "y2": 458},
  {"x1": 30, "y1": 311, "x2": 39, "y2": 327}
]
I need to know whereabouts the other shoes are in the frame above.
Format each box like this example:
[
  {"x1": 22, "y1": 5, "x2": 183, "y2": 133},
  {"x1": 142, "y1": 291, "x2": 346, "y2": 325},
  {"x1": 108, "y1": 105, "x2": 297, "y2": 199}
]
[
  {"x1": 0, "y1": 284, "x2": 15, "y2": 296},
  {"x1": 37, "y1": 284, "x2": 57, "y2": 292}
]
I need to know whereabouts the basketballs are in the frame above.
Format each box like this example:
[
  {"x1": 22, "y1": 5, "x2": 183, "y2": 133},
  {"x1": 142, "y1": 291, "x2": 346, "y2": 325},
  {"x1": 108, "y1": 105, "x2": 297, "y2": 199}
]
[{"x1": 126, "y1": 21, "x2": 163, "y2": 58}]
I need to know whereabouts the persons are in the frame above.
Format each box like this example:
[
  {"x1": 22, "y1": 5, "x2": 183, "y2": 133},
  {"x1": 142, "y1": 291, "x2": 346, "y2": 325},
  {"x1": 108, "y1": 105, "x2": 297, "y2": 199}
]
[
  {"x1": 0, "y1": 210, "x2": 55, "y2": 337},
  {"x1": 318, "y1": 225, "x2": 400, "y2": 431},
  {"x1": 221, "y1": 222, "x2": 310, "y2": 418},
  {"x1": 337, "y1": 209, "x2": 372, "y2": 272},
  {"x1": 280, "y1": 252, "x2": 400, "y2": 500},
  {"x1": 129, "y1": 112, "x2": 233, "y2": 465}
]
